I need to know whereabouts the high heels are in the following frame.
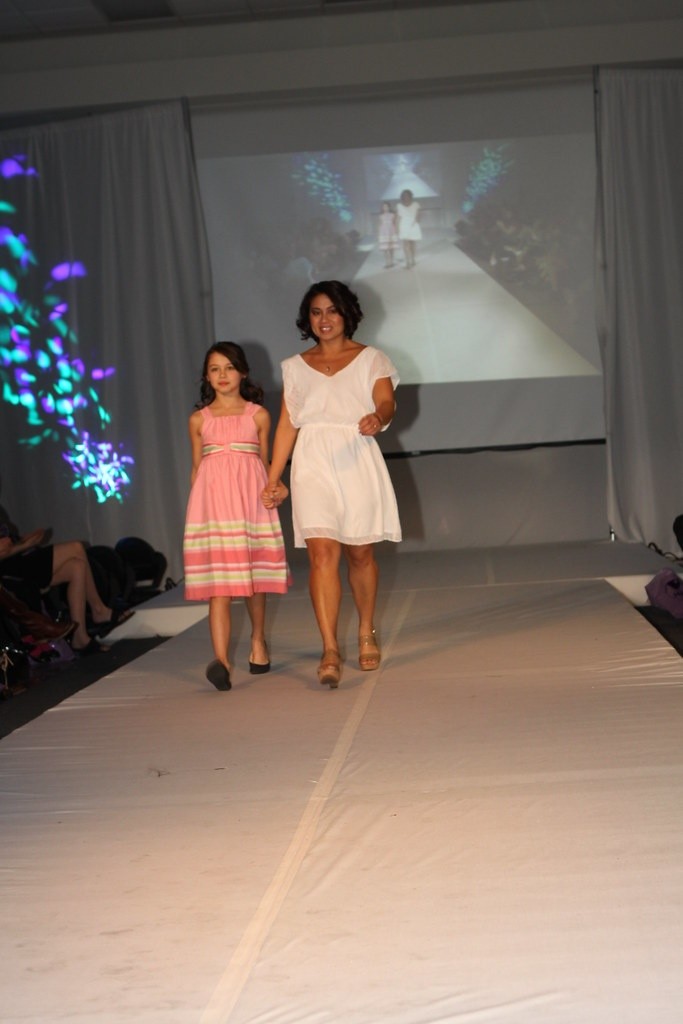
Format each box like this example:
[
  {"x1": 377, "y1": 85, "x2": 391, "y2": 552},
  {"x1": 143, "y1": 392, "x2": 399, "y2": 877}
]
[
  {"x1": 357, "y1": 634, "x2": 381, "y2": 671},
  {"x1": 317, "y1": 650, "x2": 342, "y2": 689}
]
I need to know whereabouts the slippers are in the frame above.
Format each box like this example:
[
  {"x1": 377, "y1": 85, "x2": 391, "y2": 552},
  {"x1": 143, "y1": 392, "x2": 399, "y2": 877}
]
[
  {"x1": 98, "y1": 609, "x2": 135, "y2": 637},
  {"x1": 73, "y1": 639, "x2": 110, "y2": 654}
]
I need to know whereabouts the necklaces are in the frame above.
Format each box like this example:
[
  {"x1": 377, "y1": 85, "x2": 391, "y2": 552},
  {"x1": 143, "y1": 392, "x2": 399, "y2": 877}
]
[{"x1": 326, "y1": 366, "x2": 330, "y2": 372}]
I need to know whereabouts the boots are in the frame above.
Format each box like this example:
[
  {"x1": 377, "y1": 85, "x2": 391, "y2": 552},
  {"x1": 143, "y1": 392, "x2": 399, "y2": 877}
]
[{"x1": 0, "y1": 586, "x2": 75, "y2": 642}]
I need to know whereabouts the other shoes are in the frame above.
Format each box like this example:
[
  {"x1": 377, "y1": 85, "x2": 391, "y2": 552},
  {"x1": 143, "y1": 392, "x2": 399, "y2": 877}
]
[
  {"x1": 206, "y1": 659, "x2": 232, "y2": 691},
  {"x1": 249, "y1": 639, "x2": 271, "y2": 674}
]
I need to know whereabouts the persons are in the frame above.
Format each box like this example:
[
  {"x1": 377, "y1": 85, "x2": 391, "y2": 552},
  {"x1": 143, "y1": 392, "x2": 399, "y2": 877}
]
[
  {"x1": 455, "y1": 164, "x2": 604, "y2": 369},
  {"x1": 184, "y1": 340, "x2": 289, "y2": 692},
  {"x1": 1, "y1": 505, "x2": 136, "y2": 653},
  {"x1": 395, "y1": 190, "x2": 422, "y2": 270},
  {"x1": 378, "y1": 201, "x2": 397, "y2": 269},
  {"x1": 262, "y1": 279, "x2": 400, "y2": 689},
  {"x1": 0, "y1": 605, "x2": 56, "y2": 696},
  {"x1": 248, "y1": 209, "x2": 361, "y2": 339},
  {"x1": 0, "y1": 586, "x2": 78, "y2": 641}
]
